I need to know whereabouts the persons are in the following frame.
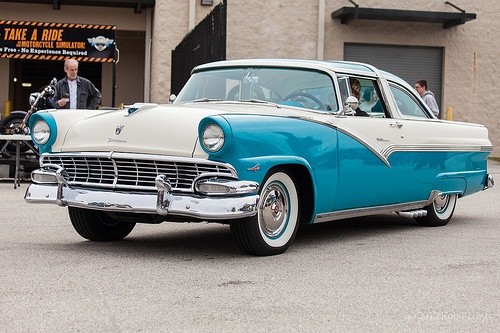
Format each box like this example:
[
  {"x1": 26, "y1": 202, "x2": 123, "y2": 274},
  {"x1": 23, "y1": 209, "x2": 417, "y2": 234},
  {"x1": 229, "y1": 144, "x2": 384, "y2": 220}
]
[
  {"x1": 415, "y1": 80, "x2": 439, "y2": 116},
  {"x1": 340, "y1": 77, "x2": 369, "y2": 117},
  {"x1": 48, "y1": 59, "x2": 102, "y2": 110}
]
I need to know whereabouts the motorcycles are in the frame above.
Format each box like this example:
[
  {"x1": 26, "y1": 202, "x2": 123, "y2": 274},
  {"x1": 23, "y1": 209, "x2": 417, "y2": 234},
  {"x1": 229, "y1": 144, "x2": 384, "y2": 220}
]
[{"x1": 0, "y1": 76, "x2": 122, "y2": 152}]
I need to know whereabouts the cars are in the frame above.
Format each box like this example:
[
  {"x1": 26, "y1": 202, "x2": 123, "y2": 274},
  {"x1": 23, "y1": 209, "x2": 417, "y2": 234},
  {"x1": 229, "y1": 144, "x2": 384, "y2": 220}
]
[{"x1": 23, "y1": 58, "x2": 496, "y2": 255}]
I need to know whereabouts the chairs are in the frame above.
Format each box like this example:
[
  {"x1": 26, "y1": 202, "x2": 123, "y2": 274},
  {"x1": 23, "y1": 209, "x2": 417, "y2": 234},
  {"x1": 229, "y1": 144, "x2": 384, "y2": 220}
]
[{"x1": 362, "y1": 89, "x2": 383, "y2": 112}]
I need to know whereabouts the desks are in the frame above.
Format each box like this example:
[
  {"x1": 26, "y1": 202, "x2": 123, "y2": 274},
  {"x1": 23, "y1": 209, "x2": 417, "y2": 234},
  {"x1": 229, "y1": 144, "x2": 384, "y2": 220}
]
[{"x1": 0, "y1": 133, "x2": 32, "y2": 189}]
